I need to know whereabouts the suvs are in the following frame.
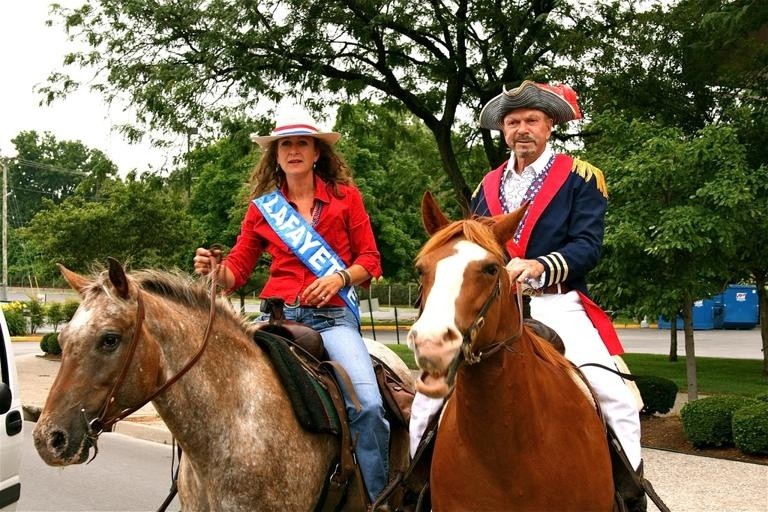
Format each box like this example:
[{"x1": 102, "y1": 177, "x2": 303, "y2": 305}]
[{"x1": 0, "y1": 304, "x2": 25, "y2": 512}]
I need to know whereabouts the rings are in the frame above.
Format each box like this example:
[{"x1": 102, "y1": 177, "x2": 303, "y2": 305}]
[{"x1": 319, "y1": 294, "x2": 325, "y2": 300}]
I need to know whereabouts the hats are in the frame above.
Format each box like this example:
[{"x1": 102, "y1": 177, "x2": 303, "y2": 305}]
[
  {"x1": 249, "y1": 105, "x2": 342, "y2": 149},
  {"x1": 478, "y1": 79, "x2": 583, "y2": 131}
]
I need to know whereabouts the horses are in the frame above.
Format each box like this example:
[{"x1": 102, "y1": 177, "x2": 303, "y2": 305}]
[
  {"x1": 406, "y1": 189, "x2": 615, "y2": 512},
  {"x1": 32, "y1": 256, "x2": 409, "y2": 512}
]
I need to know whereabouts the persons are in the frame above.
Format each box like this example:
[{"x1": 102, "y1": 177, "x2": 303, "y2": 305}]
[
  {"x1": 194, "y1": 106, "x2": 391, "y2": 512},
  {"x1": 399, "y1": 80, "x2": 647, "y2": 512}
]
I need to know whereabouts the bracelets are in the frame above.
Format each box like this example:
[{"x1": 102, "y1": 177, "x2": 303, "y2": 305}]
[{"x1": 335, "y1": 269, "x2": 351, "y2": 290}]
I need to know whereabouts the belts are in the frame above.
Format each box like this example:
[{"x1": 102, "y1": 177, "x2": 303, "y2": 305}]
[
  {"x1": 276, "y1": 296, "x2": 341, "y2": 308},
  {"x1": 540, "y1": 281, "x2": 569, "y2": 295}
]
